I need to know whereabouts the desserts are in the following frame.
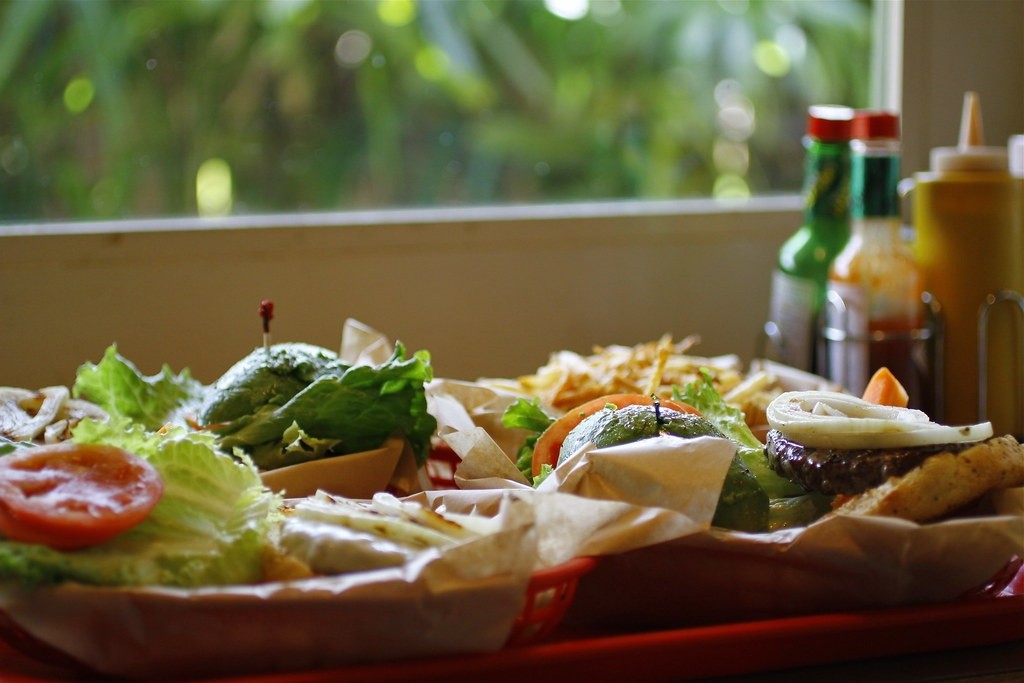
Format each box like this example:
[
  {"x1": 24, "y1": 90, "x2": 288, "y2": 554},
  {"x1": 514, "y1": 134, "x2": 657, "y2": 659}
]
[{"x1": 197, "y1": 342, "x2": 771, "y2": 534}]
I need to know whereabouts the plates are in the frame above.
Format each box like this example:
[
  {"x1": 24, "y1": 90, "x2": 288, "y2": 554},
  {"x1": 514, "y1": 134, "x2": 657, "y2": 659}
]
[
  {"x1": 254, "y1": 436, "x2": 405, "y2": 502},
  {"x1": 575, "y1": 542, "x2": 1020, "y2": 630},
  {"x1": 2, "y1": 572, "x2": 526, "y2": 674},
  {"x1": 1, "y1": 598, "x2": 1024, "y2": 683}
]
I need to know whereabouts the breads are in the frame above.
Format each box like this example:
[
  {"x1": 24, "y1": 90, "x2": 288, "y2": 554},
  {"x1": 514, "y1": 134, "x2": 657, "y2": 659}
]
[{"x1": 829, "y1": 436, "x2": 1024, "y2": 520}]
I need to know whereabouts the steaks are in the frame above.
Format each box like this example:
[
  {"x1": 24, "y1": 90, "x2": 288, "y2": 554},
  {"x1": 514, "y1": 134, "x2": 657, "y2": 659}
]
[{"x1": 764, "y1": 426, "x2": 973, "y2": 495}]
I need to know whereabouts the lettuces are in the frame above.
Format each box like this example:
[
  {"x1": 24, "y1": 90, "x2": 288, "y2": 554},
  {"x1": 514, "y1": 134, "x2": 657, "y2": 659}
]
[
  {"x1": 501, "y1": 367, "x2": 817, "y2": 535},
  {"x1": 0, "y1": 343, "x2": 439, "y2": 593}
]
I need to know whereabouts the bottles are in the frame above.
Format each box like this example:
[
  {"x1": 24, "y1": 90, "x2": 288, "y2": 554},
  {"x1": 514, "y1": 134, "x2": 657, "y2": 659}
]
[
  {"x1": 761, "y1": 105, "x2": 855, "y2": 377},
  {"x1": 819, "y1": 108, "x2": 928, "y2": 407},
  {"x1": 907, "y1": 84, "x2": 1024, "y2": 441}
]
